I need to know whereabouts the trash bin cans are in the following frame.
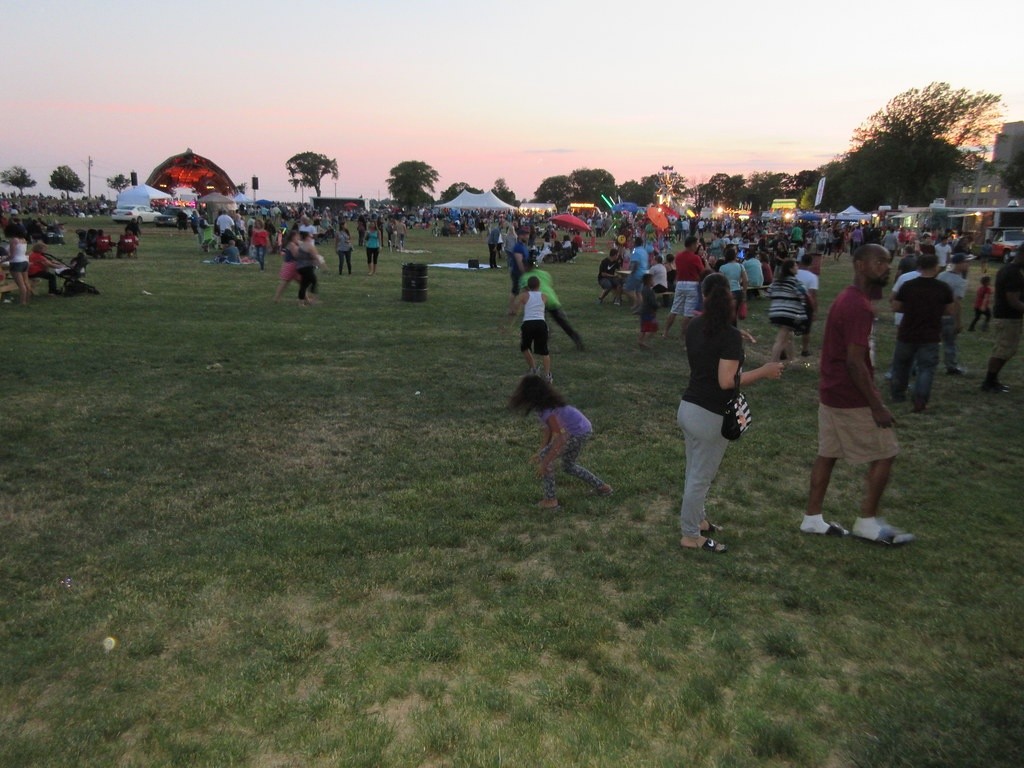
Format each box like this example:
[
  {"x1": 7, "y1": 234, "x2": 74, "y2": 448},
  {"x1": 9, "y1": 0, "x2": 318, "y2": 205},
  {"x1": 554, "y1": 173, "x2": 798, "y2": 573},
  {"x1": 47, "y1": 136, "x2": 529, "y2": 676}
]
[{"x1": 402, "y1": 264, "x2": 428, "y2": 301}]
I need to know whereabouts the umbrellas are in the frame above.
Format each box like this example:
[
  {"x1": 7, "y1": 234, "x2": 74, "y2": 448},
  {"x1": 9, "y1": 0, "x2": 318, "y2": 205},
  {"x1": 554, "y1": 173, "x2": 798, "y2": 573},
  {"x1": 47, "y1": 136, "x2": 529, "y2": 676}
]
[
  {"x1": 612, "y1": 202, "x2": 680, "y2": 228},
  {"x1": 345, "y1": 202, "x2": 358, "y2": 207},
  {"x1": 548, "y1": 213, "x2": 592, "y2": 237}
]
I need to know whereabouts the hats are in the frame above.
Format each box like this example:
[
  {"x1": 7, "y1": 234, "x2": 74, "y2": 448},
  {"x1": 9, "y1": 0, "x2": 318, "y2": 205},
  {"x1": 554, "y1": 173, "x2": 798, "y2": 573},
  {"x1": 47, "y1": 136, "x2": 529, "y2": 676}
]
[{"x1": 952, "y1": 253, "x2": 971, "y2": 263}]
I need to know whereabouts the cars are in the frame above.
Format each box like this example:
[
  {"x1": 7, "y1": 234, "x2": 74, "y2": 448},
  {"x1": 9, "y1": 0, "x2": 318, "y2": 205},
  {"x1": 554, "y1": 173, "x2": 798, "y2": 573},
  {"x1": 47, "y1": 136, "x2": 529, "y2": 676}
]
[{"x1": 111, "y1": 205, "x2": 162, "y2": 224}]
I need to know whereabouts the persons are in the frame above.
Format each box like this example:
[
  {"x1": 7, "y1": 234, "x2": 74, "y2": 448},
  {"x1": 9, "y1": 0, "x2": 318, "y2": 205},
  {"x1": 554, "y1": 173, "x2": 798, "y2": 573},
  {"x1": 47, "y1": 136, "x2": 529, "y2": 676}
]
[
  {"x1": 677, "y1": 273, "x2": 786, "y2": 554},
  {"x1": 509, "y1": 373, "x2": 613, "y2": 509},
  {"x1": 800, "y1": 244, "x2": 914, "y2": 547},
  {"x1": 0, "y1": 204, "x2": 1024, "y2": 413}
]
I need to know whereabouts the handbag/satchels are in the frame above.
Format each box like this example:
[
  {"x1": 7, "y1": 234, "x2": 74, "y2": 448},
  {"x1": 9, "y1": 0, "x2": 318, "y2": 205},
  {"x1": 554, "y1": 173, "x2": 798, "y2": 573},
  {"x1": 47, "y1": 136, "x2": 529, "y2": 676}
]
[
  {"x1": 738, "y1": 301, "x2": 747, "y2": 319},
  {"x1": 721, "y1": 363, "x2": 752, "y2": 441},
  {"x1": 640, "y1": 316, "x2": 659, "y2": 332},
  {"x1": 350, "y1": 246, "x2": 354, "y2": 251},
  {"x1": 365, "y1": 234, "x2": 369, "y2": 241}
]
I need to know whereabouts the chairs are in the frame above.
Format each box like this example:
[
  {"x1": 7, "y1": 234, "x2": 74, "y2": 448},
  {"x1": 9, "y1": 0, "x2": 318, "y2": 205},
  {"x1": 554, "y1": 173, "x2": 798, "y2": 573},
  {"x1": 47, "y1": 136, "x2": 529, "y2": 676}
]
[
  {"x1": 652, "y1": 281, "x2": 674, "y2": 308},
  {"x1": 271, "y1": 229, "x2": 283, "y2": 255},
  {"x1": 530, "y1": 235, "x2": 597, "y2": 267},
  {"x1": 76, "y1": 229, "x2": 138, "y2": 259}
]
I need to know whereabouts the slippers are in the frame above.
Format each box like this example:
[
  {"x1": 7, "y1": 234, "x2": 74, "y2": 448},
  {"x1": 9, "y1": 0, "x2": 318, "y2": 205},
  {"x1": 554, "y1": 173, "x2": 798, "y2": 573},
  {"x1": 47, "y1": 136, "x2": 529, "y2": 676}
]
[
  {"x1": 852, "y1": 526, "x2": 915, "y2": 546},
  {"x1": 799, "y1": 520, "x2": 850, "y2": 537},
  {"x1": 700, "y1": 520, "x2": 724, "y2": 532},
  {"x1": 687, "y1": 538, "x2": 728, "y2": 554}
]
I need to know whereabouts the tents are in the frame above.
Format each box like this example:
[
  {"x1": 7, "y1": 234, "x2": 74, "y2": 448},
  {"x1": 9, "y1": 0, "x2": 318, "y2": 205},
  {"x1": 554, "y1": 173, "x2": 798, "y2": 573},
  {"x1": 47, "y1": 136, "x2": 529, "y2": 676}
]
[
  {"x1": 796, "y1": 213, "x2": 821, "y2": 224},
  {"x1": 434, "y1": 190, "x2": 557, "y2": 217},
  {"x1": 830, "y1": 205, "x2": 873, "y2": 226},
  {"x1": 115, "y1": 184, "x2": 270, "y2": 225}
]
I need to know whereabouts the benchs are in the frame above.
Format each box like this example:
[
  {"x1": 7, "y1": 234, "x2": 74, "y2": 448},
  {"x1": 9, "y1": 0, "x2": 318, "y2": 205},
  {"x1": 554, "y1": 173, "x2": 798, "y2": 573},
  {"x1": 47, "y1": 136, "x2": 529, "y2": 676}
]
[
  {"x1": 0, "y1": 277, "x2": 40, "y2": 298},
  {"x1": 742, "y1": 284, "x2": 770, "y2": 289}
]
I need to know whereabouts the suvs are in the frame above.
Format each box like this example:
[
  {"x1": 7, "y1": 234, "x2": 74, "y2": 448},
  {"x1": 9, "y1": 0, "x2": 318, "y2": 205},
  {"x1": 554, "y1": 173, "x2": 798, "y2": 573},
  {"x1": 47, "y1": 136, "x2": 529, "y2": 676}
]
[{"x1": 154, "y1": 206, "x2": 194, "y2": 227}]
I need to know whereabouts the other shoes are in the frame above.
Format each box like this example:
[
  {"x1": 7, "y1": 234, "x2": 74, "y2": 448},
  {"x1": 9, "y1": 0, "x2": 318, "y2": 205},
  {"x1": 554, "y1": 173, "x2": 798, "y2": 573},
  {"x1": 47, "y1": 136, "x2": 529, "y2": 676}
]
[
  {"x1": 982, "y1": 382, "x2": 1011, "y2": 393},
  {"x1": 914, "y1": 399, "x2": 927, "y2": 410},
  {"x1": 984, "y1": 268, "x2": 987, "y2": 273},
  {"x1": 538, "y1": 500, "x2": 561, "y2": 512},
  {"x1": 588, "y1": 486, "x2": 613, "y2": 496},
  {"x1": 892, "y1": 391, "x2": 906, "y2": 400},
  {"x1": 781, "y1": 349, "x2": 788, "y2": 359},
  {"x1": 947, "y1": 367, "x2": 967, "y2": 375},
  {"x1": 368, "y1": 271, "x2": 376, "y2": 275},
  {"x1": 526, "y1": 366, "x2": 540, "y2": 376},
  {"x1": 801, "y1": 351, "x2": 813, "y2": 356},
  {"x1": 544, "y1": 372, "x2": 553, "y2": 384}
]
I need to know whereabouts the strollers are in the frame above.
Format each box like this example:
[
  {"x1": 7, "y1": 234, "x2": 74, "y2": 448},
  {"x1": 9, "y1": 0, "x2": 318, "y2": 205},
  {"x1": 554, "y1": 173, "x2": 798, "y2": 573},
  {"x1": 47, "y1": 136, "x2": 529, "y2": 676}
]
[
  {"x1": 42, "y1": 252, "x2": 99, "y2": 297},
  {"x1": 529, "y1": 242, "x2": 553, "y2": 268},
  {"x1": 314, "y1": 229, "x2": 331, "y2": 245}
]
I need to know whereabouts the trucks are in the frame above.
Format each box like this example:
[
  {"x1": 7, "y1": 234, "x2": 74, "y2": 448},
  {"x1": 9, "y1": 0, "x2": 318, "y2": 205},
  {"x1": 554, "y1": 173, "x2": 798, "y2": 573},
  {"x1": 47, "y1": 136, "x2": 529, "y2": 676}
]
[{"x1": 958, "y1": 207, "x2": 1024, "y2": 264}]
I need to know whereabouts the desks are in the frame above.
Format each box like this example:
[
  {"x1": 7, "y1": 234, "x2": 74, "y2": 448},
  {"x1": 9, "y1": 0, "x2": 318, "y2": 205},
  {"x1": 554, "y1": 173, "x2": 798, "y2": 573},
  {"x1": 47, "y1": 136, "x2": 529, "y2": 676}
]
[{"x1": 615, "y1": 271, "x2": 650, "y2": 283}]
